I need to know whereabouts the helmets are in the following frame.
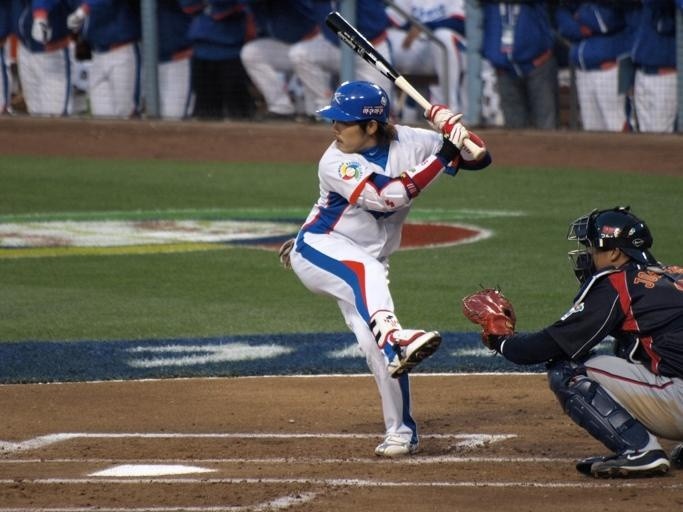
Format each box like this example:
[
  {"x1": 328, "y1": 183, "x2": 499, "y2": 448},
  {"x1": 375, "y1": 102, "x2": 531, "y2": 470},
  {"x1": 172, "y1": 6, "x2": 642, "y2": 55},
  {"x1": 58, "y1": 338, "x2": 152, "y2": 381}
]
[
  {"x1": 565, "y1": 205, "x2": 658, "y2": 290},
  {"x1": 315, "y1": 80, "x2": 390, "y2": 125}
]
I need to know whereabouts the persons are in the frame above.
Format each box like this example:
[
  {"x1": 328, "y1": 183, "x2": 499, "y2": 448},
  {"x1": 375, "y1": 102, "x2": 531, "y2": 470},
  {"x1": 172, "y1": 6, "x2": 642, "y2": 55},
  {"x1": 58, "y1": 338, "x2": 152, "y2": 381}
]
[
  {"x1": 287, "y1": 81, "x2": 492, "y2": 455},
  {"x1": 462, "y1": 208, "x2": 683, "y2": 478}
]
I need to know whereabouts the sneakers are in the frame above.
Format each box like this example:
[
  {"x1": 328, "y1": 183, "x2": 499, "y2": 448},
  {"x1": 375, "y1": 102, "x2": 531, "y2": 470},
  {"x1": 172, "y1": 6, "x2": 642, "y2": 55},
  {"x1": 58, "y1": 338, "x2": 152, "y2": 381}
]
[
  {"x1": 374, "y1": 438, "x2": 421, "y2": 457},
  {"x1": 380, "y1": 329, "x2": 441, "y2": 378},
  {"x1": 590, "y1": 448, "x2": 671, "y2": 479}
]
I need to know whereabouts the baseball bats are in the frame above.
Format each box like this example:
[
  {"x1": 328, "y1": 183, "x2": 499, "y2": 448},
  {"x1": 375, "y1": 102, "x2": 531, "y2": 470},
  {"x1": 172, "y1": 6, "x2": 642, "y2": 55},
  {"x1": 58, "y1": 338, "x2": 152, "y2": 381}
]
[{"x1": 325, "y1": 12, "x2": 486, "y2": 160}]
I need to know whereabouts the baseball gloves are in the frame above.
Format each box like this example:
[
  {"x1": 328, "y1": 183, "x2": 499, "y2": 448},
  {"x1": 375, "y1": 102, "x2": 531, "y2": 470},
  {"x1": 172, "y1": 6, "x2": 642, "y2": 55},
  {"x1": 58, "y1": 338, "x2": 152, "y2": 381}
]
[{"x1": 461, "y1": 290, "x2": 516, "y2": 352}]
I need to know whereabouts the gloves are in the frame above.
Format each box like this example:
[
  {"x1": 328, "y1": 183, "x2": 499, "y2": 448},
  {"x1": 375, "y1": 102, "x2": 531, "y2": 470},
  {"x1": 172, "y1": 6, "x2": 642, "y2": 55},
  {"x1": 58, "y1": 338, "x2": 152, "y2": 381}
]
[{"x1": 423, "y1": 103, "x2": 470, "y2": 162}]
